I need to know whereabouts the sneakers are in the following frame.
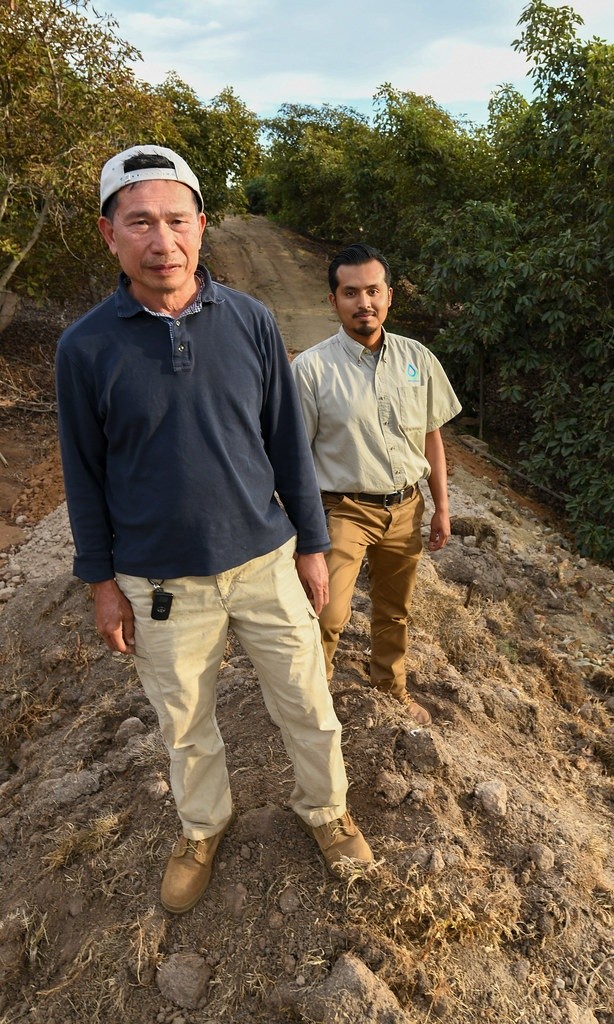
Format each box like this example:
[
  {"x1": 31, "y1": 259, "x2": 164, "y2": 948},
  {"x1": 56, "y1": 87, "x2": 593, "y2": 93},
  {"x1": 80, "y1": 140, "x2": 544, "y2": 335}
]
[
  {"x1": 394, "y1": 691, "x2": 432, "y2": 727},
  {"x1": 160, "y1": 807, "x2": 233, "y2": 913},
  {"x1": 295, "y1": 811, "x2": 376, "y2": 881}
]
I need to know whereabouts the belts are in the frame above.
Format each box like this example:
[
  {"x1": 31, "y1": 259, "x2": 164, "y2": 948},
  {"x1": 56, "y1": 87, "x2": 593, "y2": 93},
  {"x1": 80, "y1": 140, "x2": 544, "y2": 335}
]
[{"x1": 323, "y1": 482, "x2": 419, "y2": 506}]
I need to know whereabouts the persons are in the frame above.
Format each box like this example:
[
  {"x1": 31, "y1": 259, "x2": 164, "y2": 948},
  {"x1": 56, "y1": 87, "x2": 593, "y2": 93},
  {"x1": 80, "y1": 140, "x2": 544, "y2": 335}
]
[
  {"x1": 290, "y1": 244, "x2": 462, "y2": 728},
  {"x1": 56, "y1": 145, "x2": 374, "y2": 914}
]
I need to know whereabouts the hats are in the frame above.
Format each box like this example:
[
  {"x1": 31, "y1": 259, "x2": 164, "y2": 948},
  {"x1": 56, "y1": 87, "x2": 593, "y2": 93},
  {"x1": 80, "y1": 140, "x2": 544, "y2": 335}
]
[{"x1": 101, "y1": 143, "x2": 204, "y2": 213}]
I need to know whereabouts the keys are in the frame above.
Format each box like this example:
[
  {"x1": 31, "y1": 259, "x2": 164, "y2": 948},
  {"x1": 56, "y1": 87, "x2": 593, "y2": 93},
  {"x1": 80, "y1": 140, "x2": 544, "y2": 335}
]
[{"x1": 148, "y1": 578, "x2": 173, "y2": 620}]
